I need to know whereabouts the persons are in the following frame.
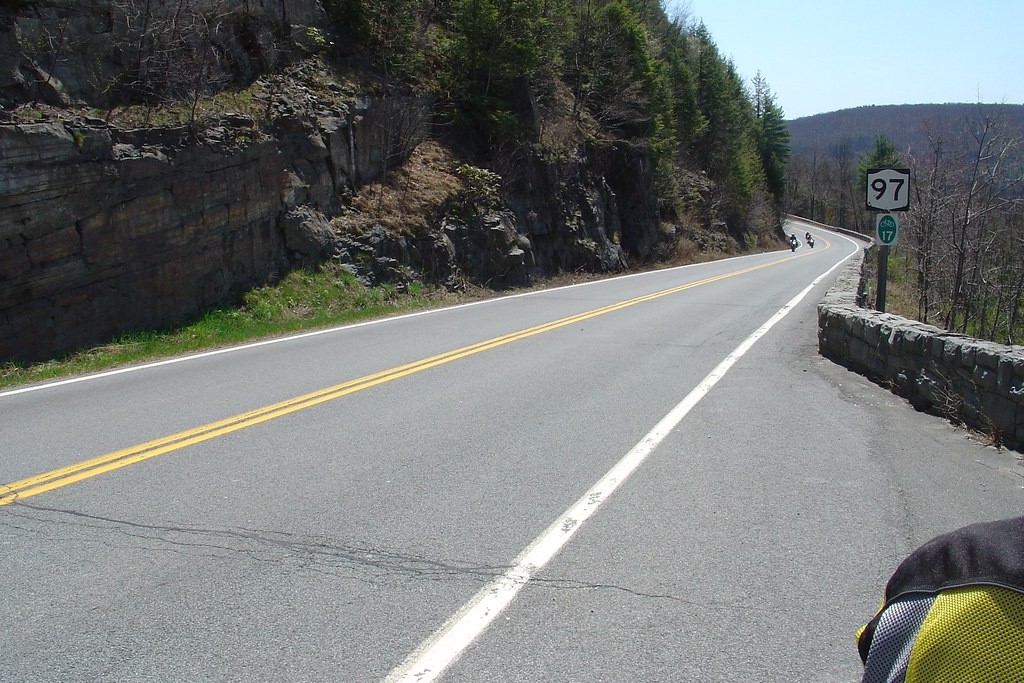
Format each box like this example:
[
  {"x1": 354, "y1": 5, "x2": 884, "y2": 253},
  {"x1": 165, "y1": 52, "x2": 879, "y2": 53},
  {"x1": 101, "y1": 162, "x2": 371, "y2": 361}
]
[
  {"x1": 789, "y1": 233, "x2": 798, "y2": 248},
  {"x1": 805, "y1": 231, "x2": 815, "y2": 244},
  {"x1": 855, "y1": 517, "x2": 1024, "y2": 683}
]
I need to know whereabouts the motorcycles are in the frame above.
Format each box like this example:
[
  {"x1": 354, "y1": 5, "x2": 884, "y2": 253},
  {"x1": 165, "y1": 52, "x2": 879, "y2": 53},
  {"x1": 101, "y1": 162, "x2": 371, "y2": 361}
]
[
  {"x1": 790, "y1": 239, "x2": 796, "y2": 252},
  {"x1": 807, "y1": 235, "x2": 814, "y2": 248}
]
